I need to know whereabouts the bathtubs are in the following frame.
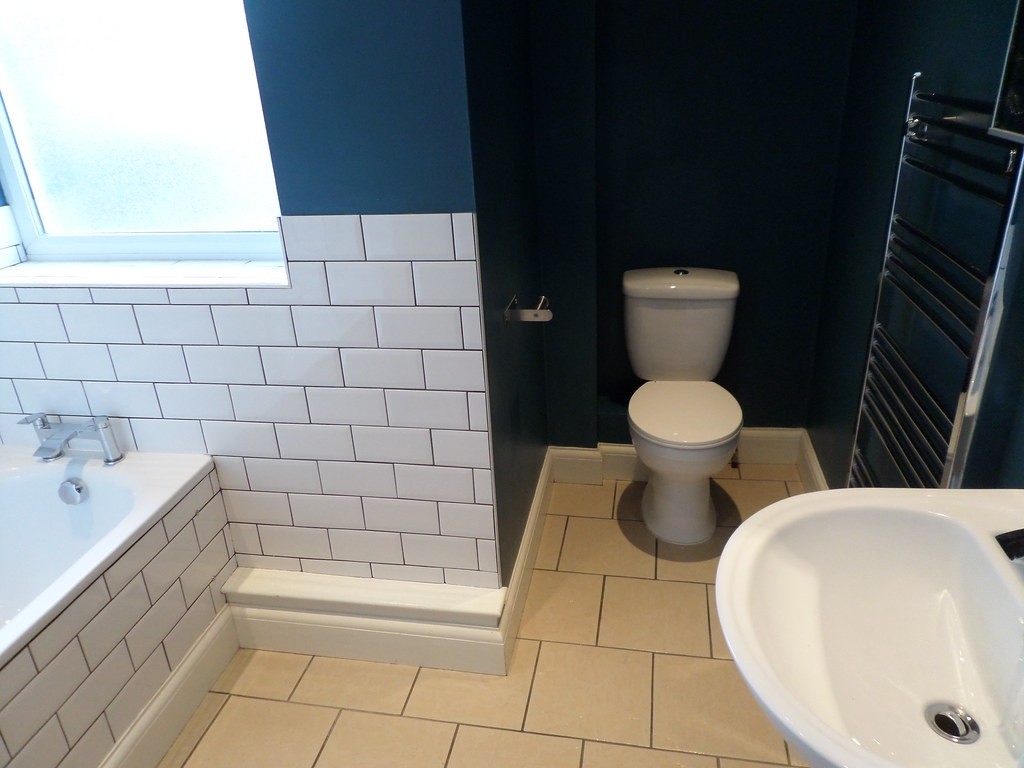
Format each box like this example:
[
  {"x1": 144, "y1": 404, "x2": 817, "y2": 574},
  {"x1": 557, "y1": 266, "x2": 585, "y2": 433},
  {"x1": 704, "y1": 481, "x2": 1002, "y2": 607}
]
[{"x1": 0, "y1": 443, "x2": 240, "y2": 768}]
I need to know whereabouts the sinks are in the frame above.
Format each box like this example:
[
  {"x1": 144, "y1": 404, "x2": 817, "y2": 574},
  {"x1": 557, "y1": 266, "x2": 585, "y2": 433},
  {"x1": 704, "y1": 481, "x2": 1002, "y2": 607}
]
[{"x1": 711, "y1": 483, "x2": 1024, "y2": 768}]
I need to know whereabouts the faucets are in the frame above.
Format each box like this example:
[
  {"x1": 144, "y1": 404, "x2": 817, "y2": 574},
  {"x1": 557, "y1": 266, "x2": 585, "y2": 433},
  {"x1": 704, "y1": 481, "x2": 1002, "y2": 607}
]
[{"x1": 16, "y1": 410, "x2": 95, "y2": 465}]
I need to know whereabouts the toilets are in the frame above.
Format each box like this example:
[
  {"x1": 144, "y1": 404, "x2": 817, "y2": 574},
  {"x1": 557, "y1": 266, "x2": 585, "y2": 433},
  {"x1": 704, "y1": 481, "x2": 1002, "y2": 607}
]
[{"x1": 618, "y1": 263, "x2": 746, "y2": 547}]
